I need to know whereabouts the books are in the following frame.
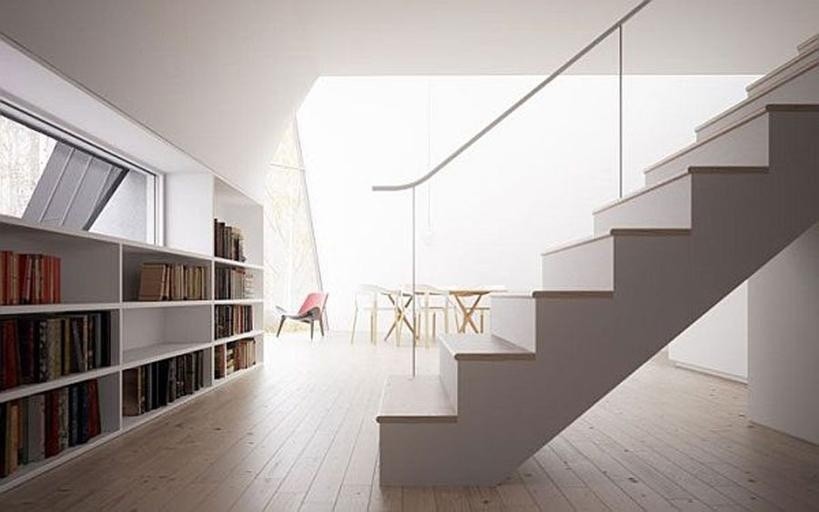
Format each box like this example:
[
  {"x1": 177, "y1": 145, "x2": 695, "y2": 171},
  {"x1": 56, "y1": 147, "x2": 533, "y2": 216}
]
[
  {"x1": 137, "y1": 261, "x2": 209, "y2": 301},
  {"x1": 1, "y1": 250, "x2": 61, "y2": 305},
  {"x1": 123, "y1": 350, "x2": 204, "y2": 417},
  {"x1": 215, "y1": 262, "x2": 256, "y2": 300},
  {"x1": 1, "y1": 310, "x2": 111, "y2": 390},
  {"x1": 214, "y1": 304, "x2": 253, "y2": 340},
  {"x1": 214, "y1": 218, "x2": 246, "y2": 262},
  {"x1": 214, "y1": 337, "x2": 255, "y2": 379},
  {"x1": 1, "y1": 378, "x2": 102, "y2": 480}
]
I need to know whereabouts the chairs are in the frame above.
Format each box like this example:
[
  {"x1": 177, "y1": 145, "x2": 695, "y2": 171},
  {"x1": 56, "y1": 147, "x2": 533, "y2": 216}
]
[
  {"x1": 275, "y1": 291, "x2": 329, "y2": 340},
  {"x1": 351, "y1": 284, "x2": 449, "y2": 347}
]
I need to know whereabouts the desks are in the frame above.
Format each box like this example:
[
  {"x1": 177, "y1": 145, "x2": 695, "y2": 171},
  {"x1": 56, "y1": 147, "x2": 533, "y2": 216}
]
[{"x1": 380, "y1": 290, "x2": 491, "y2": 343}]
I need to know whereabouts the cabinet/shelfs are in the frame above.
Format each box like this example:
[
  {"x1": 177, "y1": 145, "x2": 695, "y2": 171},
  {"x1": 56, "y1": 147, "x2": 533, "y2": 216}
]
[
  {"x1": 214, "y1": 175, "x2": 264, "y2": 387},
  {"x1": 1, "y1": 214, "x2": 210, "y2": 494}
]
